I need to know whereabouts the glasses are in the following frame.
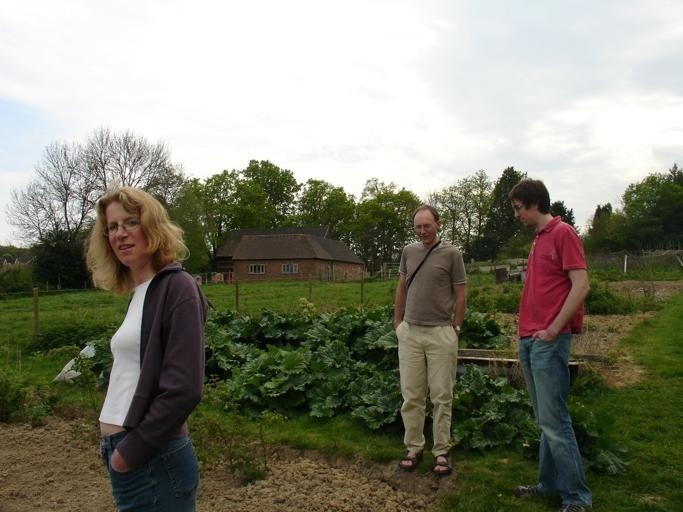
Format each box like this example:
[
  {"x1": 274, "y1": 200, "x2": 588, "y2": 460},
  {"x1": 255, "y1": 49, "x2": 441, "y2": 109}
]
[
  {"x1": 102, "y1": 219, "x2": 141, "y2": 236},
  {"x1": 512, "y1": 204, "x2": 526, "y2": 211}
]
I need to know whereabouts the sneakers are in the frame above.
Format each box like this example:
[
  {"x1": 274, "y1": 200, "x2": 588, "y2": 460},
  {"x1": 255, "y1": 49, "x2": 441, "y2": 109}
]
[
  {"x1": 514, "y1": 485, "x2": 561, "y2": 506},
  {"x1": 557, "y1": 504, "x2": 588, "y2": 512}
]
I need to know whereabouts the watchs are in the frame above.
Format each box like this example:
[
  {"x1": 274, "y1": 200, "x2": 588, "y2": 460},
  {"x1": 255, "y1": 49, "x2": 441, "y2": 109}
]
[{"x1": 453, "y1": 324, "x2": 461, "y2": 331}]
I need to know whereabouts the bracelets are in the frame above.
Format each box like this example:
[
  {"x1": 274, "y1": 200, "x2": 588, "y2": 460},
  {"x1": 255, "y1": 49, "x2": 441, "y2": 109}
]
[{"x1": 545, "y1": 329, "x2": 555, "y2": 339}]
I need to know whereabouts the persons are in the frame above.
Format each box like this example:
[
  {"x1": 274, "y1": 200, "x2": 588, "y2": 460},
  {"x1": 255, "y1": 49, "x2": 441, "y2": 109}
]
[
  {"x1": 510, "y1": 179, "x2": 594, "y2": 512},
  {"x1": 86, "y1": 188, "x2": 209, "y2": 512},
  {"x1": 392, "y1": 204, "x2": 467, "y2": 476}
]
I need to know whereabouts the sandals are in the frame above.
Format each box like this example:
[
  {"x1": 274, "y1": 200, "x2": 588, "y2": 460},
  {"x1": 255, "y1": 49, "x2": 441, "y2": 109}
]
[
  {"x1": 398, "y1": 449, "x2": 424, "y2": 471},
  {"x1": 431, "y1": 453, "x2": 452, "y2": 475}
]
